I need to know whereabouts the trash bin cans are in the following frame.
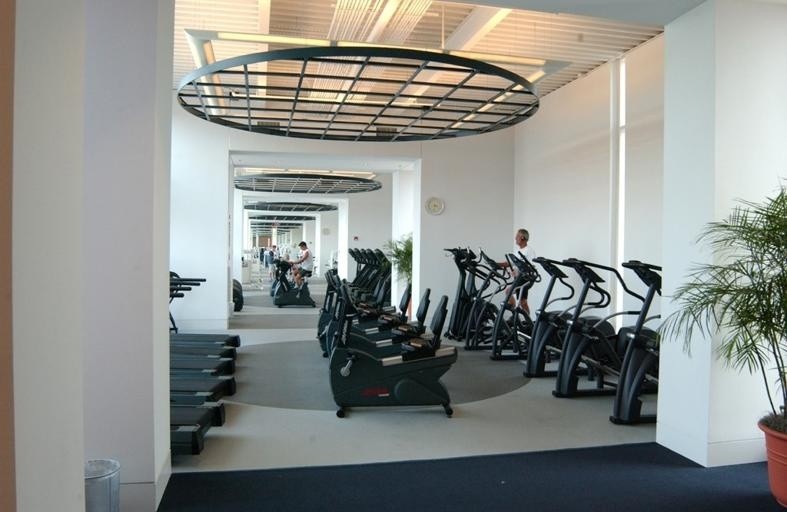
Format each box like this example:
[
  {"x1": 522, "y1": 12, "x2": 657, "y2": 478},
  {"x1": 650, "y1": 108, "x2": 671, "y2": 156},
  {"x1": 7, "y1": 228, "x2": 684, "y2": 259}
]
[{"x1": 241, "y1": 265, "x2": 251, "y2": 284}]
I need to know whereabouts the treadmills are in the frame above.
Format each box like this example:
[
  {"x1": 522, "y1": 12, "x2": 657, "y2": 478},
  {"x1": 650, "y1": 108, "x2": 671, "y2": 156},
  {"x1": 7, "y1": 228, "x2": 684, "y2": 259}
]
[{"x1": 168, "y1": 271, "x2": 241, "y2": 454}]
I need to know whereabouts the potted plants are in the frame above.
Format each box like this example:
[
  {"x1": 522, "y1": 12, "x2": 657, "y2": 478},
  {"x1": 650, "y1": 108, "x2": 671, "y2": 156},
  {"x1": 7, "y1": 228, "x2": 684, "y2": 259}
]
[
  {"x1": 649, "y1": 177, "x2": 787, "y2": 508},
  {"x1": 381, "y1": 236, "x2": 412, "y2": 320}
]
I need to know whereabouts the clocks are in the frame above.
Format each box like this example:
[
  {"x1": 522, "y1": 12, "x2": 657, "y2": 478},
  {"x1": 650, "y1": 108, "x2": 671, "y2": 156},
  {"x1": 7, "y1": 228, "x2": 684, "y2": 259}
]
[{"x1": 425, "y1": 196, "x2": 444, "y2": 216}]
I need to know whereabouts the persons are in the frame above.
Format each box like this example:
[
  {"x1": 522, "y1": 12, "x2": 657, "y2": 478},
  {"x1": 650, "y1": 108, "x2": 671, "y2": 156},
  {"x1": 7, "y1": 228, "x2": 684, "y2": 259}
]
[
  {"x1": 266, "y1": 242, "x2": 313, "y2": 288},
  {"x1": 498, "y1": 229, "x2": 536, "y2": 325}
]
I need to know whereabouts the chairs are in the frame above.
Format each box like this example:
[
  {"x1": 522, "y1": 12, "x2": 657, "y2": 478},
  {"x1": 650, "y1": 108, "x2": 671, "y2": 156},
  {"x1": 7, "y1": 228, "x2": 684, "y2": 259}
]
[
  {"x1": 293, "y1": 265, "x2": 311, "y2": 287},
  {"x1": 390, "y1": 288, "x2": 430, "y2": 345},
  {"x1": 403, "y1": 296, "x2": 448, "y2": 360},
  {"x1": 383, "y1": 283, "x2": 411, "y2": 331}
]
